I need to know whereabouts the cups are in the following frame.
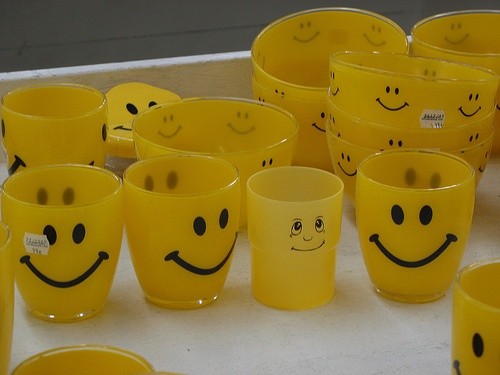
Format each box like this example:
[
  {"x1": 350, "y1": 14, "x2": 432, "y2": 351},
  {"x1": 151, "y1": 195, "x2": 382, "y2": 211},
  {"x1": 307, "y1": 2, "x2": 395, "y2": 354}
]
[
  {"x1": 122, "y1": 154, "x2": 241, "y2": 311},
  {"x1": 245, "y1": 165, "x2": 344, "y2": 311},
  {"x1": 10, "y1": 344, "x2": 156, "y2": 375},
  {"x1": 0, "y1": 82, "x2": 108, "y2": 177},
  {"x1": 451, "y1": 256, "x2": 500, "y2": 375},
  {"x1": 0, "y1": 222, "x2": 16, "y2": 375},
  {"x1": 355, "y1": 147, "x2": 476, "y2": 303},
  {"x1": 0, "y1": 163, "x2": 124, "y2": 324}
]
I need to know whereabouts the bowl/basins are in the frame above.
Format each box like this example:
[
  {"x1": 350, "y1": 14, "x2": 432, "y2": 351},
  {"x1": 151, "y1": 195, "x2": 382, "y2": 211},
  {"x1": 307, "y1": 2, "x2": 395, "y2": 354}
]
[
  {"x1": 132, "y1": 96, "x2": 299, "y2": 231},
  {"x1": 250, "y1": 7, "x2": 500, "y2": 206}
]
